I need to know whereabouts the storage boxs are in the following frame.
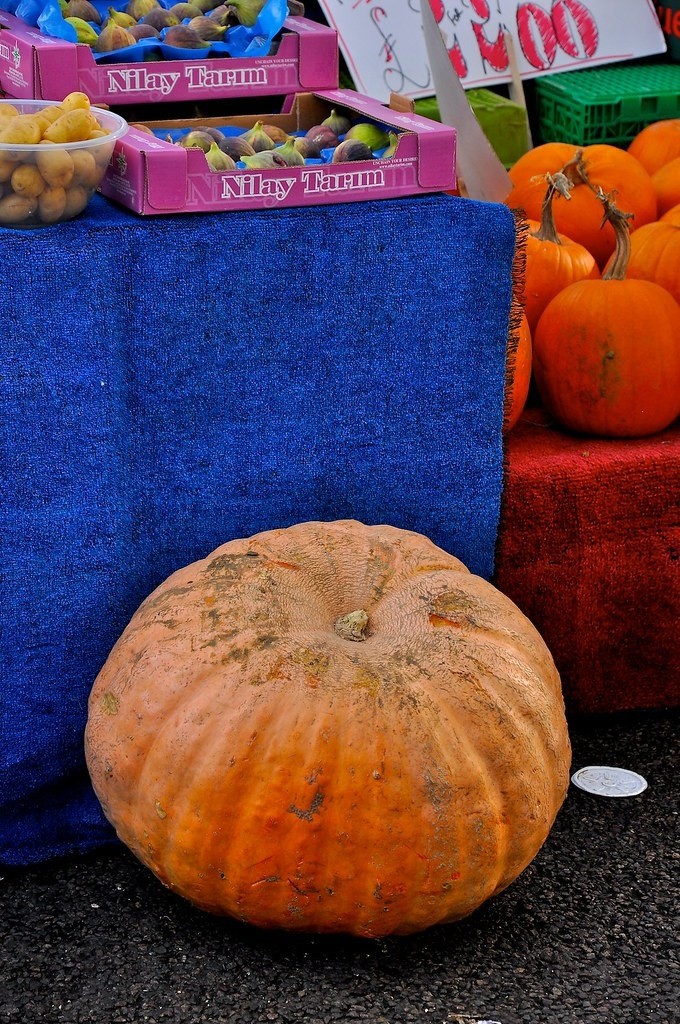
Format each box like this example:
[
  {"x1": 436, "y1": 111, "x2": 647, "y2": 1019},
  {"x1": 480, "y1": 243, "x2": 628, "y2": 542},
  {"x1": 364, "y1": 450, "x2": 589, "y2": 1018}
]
[
  {"x1": 0, "y1": 0, "x2": 306, "y2": 16},
  {"x1": 530, "y1": 64, "x2": 680, "y2": 152},
  {"x1": 97, "y1": 88, "x2": 457, "y2": 218},
  {"x1": 412, "y1": 90, "x2": 530, "y2": 171},
  {"x1": 0, "y1": 15, "x2": 339, "y2": 105}
]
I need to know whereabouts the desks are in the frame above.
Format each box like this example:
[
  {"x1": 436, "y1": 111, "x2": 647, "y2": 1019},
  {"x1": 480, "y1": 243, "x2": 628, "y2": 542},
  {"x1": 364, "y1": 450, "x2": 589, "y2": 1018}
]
[
  {"x1": 0, "y1": 193, "x2": 528, "y2": 810},
  {"x1": 499, "y1": 412, "x2": 680, "y2": 721}
]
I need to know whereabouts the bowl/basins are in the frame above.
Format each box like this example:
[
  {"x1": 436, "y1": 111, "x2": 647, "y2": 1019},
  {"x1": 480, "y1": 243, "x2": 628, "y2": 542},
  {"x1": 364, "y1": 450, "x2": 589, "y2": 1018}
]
[{"x1": 0, "y1": 100, "x2": 129, "y2": 228}]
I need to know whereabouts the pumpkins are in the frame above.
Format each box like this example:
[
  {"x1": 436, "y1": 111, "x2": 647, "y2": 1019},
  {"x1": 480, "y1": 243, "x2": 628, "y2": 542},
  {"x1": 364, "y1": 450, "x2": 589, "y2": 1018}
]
[
  {"x1": 496, "y1": 118, "x2": 680, "y2": 442},
  {"x1": 84, "y1": 518, "x2": 573, "y2": 940}
]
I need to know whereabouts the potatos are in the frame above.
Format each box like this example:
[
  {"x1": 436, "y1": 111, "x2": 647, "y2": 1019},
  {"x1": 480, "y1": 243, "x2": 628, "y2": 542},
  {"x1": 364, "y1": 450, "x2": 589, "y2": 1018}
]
[{"x1": 0, "y1": 91, "x2": 116, "y2": 226}]
